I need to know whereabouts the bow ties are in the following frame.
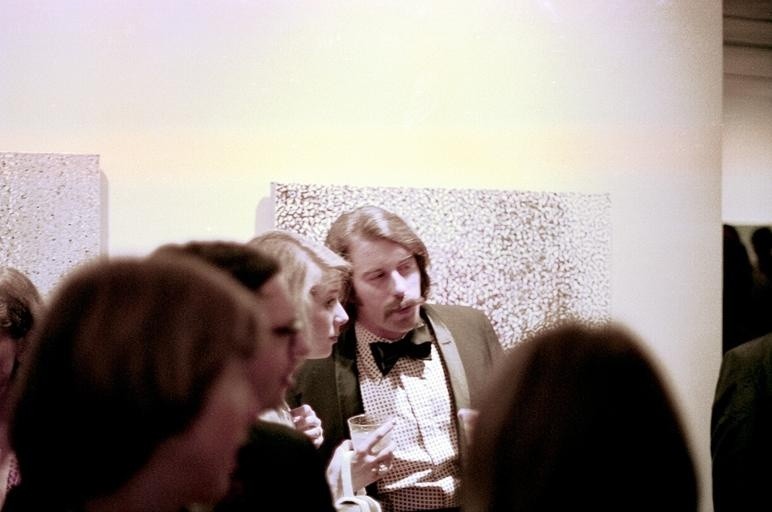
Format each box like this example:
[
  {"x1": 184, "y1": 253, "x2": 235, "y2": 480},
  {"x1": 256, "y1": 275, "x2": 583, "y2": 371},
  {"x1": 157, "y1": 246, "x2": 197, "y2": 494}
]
[{"x1": 370, "y1": 323, "x2": 434, "y2": 378}]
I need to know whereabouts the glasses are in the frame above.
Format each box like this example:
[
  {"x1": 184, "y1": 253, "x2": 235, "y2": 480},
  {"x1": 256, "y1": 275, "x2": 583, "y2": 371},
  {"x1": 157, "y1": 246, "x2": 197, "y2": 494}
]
[{"x1": 271, "y1": 318, "x2": 307, "y2": 348}]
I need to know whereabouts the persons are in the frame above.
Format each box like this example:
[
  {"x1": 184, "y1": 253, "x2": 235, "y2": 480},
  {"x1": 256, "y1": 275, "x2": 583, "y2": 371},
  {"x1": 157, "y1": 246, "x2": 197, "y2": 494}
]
[
  {"x1": 721, "y1": 222, "x2": 772, "y2": 353},
  {"x1": 1, "y1": 263, "x2": 52, "y2": 512},
  {"x1": 709, "y1": 326, "x2": 772, "y2": 511},
  {"x1": 286, "y1": 199, "x2": 513, "y2": 512},
  {"x1": 243, "y1": 228, "x2": 403, "y2": 511},
  {"x1": 751, "y1": 226, "x2": 772, "y2": 282},
  {"x1": 1, "y1": 248, "x2": 271, "y2": 511},
  {"x1": 448, "y1": 314, "x2": 702, "y2": 512},
  {"x1": 147, "y1": 239, "x2": 337, "y2": 511}
]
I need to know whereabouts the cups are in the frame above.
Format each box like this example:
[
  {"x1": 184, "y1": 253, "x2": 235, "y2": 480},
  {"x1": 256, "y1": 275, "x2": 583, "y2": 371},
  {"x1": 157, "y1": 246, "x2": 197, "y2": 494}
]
[{"x1": 346, "y1": 413, "x2": 391, "y2": 451}]
